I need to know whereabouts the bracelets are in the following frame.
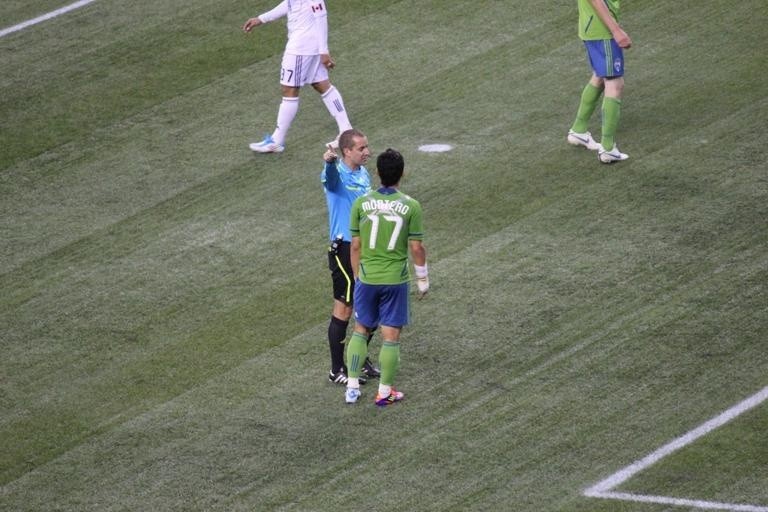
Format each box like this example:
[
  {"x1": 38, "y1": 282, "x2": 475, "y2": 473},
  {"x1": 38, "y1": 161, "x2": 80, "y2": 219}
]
[{"x1": 414, "y1": 264, "x2": 427, "y2": 278}]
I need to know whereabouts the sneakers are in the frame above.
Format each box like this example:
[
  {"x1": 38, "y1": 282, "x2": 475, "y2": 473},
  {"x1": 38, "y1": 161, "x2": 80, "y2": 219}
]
[
  {"x1": 568, "y1": 129, "x2": 600, "y2": 151},
  {"x1": 249, "y1": 137, "x2": 284, "y2": 153},
  {"x1": 598, "y1": 143, "x2": 629, "y2": 163},
  {"x1": 328, "y1": 356, "x2": 380, "y2": 403},
  {"x1": 375, "y1": 388, "x2": 404, "y2": 405},
  {"x1": 326, "y1": 134, "x2": 340, "y2": 149}
]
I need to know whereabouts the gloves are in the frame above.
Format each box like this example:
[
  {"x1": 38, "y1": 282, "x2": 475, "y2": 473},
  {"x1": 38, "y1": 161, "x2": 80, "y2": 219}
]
[{"x1": 416, "y1": 276, "x2": 429, "y2": 301}]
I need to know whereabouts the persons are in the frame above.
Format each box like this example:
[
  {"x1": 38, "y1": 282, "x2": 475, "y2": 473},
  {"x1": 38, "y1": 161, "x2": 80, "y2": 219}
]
[
  {"x1": 566, "y1": 0, "x2": 633, "y2": 164},
  {"x1": 240, "y1": 0, "x2": 354, "y2": 155},
  {"x1": 321, "y1": 129, "x2": 383, "y2": 385},
  {"x1": 344, "y1": 147, "x2": 430, "y2": 406}
]
